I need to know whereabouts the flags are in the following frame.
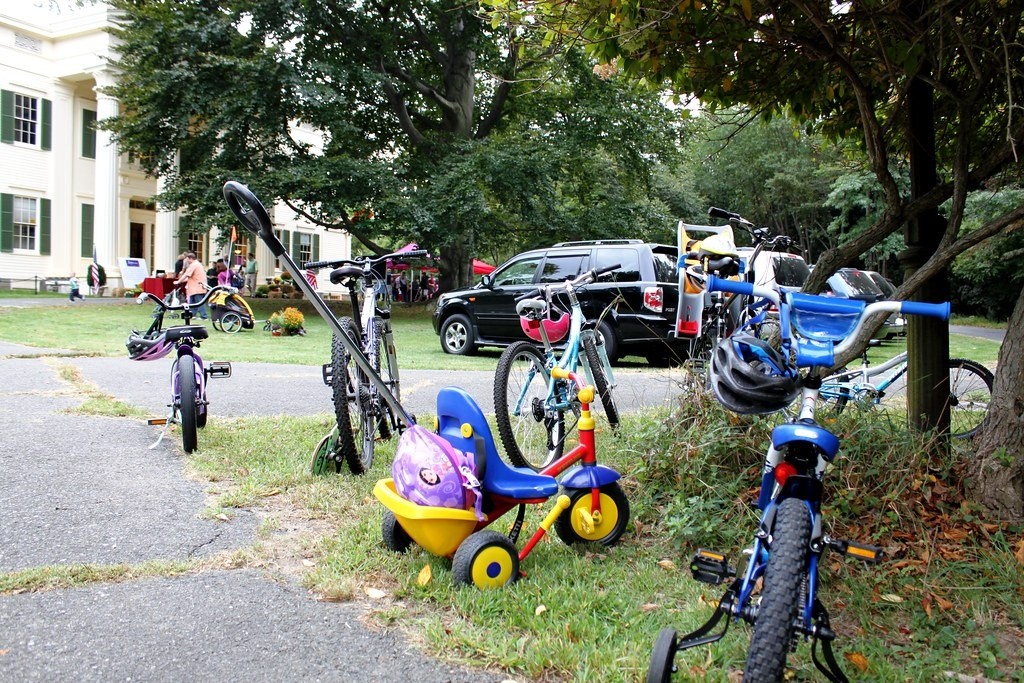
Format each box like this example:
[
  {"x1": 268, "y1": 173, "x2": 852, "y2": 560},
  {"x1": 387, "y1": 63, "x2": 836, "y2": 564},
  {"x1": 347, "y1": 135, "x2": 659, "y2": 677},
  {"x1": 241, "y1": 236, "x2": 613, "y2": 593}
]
[
  {"x1": 306, "y1": 258, "x2": 319, "y2": 290},
  {"x1": 91, "y1": 246, "x2": 99, "y2": 295}
]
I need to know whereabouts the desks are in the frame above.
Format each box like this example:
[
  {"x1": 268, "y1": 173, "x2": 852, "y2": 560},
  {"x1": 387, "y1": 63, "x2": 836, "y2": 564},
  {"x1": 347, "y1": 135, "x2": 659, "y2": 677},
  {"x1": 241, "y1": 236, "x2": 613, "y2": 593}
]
[{"x1": 141, "y1": 278, "x2": 179, "y2": 300}]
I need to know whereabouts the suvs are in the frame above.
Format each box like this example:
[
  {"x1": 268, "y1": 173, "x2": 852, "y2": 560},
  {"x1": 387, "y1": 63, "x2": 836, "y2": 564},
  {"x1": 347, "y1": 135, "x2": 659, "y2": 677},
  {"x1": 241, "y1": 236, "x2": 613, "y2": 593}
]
[{"x1": 433, "y1": 238, "x2": 908, "y2": 367}]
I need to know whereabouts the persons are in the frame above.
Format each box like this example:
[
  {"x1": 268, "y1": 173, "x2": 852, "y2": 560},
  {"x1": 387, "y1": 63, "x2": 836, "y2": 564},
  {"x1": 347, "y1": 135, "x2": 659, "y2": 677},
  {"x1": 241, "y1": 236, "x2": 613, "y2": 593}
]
[
  {"x1": 173, "y1": 251, "x2": 259, "y2": 320},
  {"x1": 390, "y1": 269, "x2": 436, "y2": 302},
  {"x1": 69, "y1": 272, "x2": 86, "y2": 303}
]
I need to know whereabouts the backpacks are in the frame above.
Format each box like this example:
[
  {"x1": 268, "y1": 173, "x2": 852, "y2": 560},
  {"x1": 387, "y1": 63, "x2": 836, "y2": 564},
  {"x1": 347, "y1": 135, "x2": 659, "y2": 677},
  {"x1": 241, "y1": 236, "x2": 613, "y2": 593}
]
[{"x1": 230, "y1": 273, "x2": 244, "y2": 289}]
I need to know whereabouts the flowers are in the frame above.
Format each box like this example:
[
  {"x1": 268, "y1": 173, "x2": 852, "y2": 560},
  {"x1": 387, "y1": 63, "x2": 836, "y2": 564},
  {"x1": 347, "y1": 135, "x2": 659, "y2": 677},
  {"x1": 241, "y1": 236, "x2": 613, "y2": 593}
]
[{"x1": 268, "y1": 307, "x2": 305, "y2": 336}]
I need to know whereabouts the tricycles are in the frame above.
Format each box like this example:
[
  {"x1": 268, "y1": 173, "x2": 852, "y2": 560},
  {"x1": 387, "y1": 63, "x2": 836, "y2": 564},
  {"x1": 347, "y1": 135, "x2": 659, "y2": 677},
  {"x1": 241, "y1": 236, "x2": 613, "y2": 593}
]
[{"x1": 219, "y1": 181, "x2": 630, "y2": 595}]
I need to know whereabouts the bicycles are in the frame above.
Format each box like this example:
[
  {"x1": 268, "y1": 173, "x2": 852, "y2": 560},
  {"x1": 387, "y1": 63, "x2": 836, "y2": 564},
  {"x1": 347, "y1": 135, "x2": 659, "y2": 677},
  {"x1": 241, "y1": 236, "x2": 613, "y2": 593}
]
[
  {"x1": 136, "y1": 286, "x2": 237, "y2": 454},
  {"x1": 645, "y1": 269, "x2": 951, "y2": 683},
  {"x1": 303, "y1": 249, "x2": 427, "y2": 475},
  {"x1": 685, "y1": 206, "x2": 994, "y2": 443},
  {"x1": 493, "y1": 263, "x2": 621, "y2": 473}
]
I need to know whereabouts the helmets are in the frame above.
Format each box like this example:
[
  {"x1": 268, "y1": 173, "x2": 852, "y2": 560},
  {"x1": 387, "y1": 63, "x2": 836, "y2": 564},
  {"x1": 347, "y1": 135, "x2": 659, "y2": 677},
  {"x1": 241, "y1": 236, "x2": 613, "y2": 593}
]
[
  {"x1": 126, "y1": 329, "x2": 175, "y2": 362},
  {"x1": 520, "y1": 313, "x2": 570, "y2": 343},
  {"x1": 708, "y1": 325, "x2": 804, "y2": 415}
]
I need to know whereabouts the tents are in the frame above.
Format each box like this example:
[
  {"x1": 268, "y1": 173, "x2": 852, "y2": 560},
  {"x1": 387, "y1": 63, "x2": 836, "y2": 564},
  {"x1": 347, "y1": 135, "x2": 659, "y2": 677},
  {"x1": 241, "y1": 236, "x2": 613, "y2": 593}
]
[{"x1": 385, "y1": 240, "x2": 497, "y2": 303}]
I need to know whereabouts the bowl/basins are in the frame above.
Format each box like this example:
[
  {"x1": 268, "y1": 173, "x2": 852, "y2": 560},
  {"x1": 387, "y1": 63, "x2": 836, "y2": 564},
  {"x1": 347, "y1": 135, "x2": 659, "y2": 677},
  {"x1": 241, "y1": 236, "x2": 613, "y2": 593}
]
[{"x1": 158, "y1": 273, "x2": 164, "y2": 278}]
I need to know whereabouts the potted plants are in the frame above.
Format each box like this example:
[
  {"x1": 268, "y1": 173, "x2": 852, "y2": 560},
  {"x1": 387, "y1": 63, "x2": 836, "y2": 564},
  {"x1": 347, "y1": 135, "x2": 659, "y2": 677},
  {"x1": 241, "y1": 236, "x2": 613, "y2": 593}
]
[
  {"x1": 124, "y1": 288, "x2": 143, "y2": 298},
  {"x1": 254, "y1": 277, "x2": 304, "y2": 299}
]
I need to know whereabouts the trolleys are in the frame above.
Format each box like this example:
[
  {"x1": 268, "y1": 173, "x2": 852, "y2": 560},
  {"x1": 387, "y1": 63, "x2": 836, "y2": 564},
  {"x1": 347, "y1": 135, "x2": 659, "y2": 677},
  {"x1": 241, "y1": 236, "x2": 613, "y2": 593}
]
[
  {"x1": 199, "y1": 281, "x2": 255, "y2": 334},
  {"x1": 151, "y1": 283, "x2": 183, "y2": 319}
]
